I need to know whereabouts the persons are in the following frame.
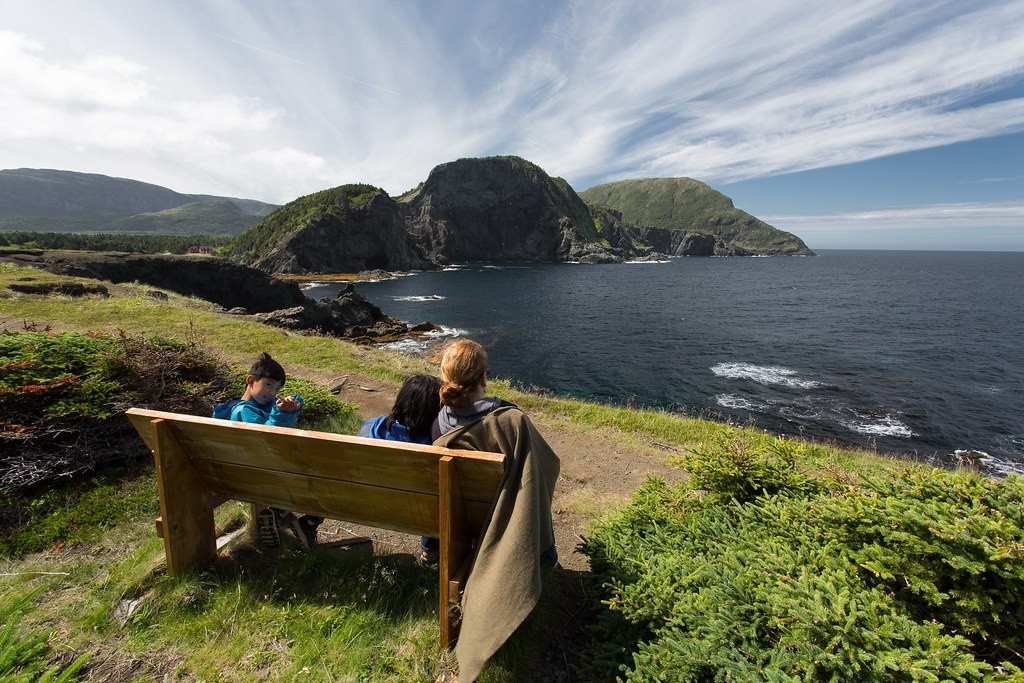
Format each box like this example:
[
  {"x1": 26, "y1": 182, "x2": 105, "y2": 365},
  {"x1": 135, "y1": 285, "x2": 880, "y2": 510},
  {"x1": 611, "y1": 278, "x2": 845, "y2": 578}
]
[
  {"x1": 352, "y1": 340, "x2": 561, "y2": 683},
  {"x1": 212, "y1": 352, "x2": 325, "y2": 550}
]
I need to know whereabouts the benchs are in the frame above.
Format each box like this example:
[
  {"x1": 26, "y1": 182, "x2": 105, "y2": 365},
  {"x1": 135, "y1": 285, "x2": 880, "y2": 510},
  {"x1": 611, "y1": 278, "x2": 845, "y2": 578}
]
[{"x1": 126, "y1": 407, "x2": 505, "y2": 648}]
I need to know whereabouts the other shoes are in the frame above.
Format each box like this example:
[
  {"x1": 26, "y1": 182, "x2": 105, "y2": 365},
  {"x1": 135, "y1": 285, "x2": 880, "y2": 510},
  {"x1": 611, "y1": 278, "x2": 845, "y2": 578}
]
[{"x1": 420, "y1": 550, "x2": 439, "y2": 561}]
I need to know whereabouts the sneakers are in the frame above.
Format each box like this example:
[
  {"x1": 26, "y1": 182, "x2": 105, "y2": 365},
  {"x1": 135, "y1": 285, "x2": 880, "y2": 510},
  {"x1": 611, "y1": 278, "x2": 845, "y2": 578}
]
[
  {"x1": 258, "y1": 508, "x2": 280, "y2": 548},
  {"x1": 288, "y1": 516, "x2": 318, "y2": 552}
]
